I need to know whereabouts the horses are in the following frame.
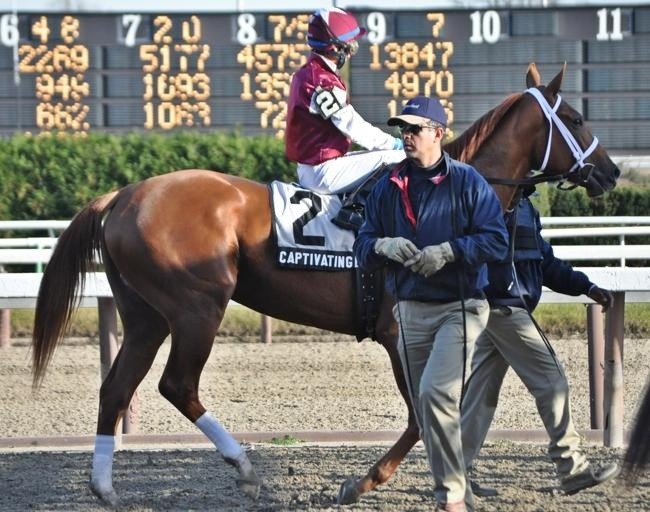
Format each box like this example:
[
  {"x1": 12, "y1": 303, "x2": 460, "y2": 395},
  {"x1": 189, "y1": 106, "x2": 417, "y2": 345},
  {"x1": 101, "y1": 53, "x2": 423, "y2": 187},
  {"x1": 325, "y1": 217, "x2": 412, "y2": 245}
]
[{"x1": 26, "y1": 61, "x2": 621, "y2": 506}]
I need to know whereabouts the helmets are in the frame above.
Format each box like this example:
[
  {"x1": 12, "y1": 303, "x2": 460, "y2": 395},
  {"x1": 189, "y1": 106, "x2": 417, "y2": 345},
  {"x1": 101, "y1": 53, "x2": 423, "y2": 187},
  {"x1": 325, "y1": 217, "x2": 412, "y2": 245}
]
[{"x1": 307, "y1": 6, "x2": 366, "y2": 51}]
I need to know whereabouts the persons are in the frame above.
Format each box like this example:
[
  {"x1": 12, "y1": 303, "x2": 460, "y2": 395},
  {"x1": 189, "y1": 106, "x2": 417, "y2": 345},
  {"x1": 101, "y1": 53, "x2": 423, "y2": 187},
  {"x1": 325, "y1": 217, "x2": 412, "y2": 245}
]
[
  {"x1": 284, "y1": 7, "x2": 408, "y2": 231},
  {"x1": 350, "y1": 96, "x2": 509, "y2": 511},
  {"x1": 458, "y1": 169, "x2": 620, "y2": 497}
]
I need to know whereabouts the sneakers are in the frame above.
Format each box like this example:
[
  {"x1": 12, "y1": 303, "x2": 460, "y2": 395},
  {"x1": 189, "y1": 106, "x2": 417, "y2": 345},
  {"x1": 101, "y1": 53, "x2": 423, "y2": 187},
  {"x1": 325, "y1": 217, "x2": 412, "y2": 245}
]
[
  {"x1": 563, "y1": 463, "x2": 620, "y2": 494},
  {"x1": 468, "y1": 479, "x2": 498, "y2": 496}
]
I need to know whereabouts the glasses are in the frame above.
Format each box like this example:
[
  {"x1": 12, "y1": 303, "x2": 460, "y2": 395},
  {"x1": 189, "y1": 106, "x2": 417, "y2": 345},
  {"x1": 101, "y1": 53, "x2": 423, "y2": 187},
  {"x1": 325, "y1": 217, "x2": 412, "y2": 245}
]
[{"x1": 399, "y1": 125, "x2": 435, "y2": 134}]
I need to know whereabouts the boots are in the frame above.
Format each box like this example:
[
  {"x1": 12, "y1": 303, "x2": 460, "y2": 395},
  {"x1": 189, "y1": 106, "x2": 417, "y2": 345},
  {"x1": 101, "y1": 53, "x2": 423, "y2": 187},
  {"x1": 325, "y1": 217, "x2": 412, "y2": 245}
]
[{"x1": 335, "y1": 162, "x2": 394, "y2": 232}]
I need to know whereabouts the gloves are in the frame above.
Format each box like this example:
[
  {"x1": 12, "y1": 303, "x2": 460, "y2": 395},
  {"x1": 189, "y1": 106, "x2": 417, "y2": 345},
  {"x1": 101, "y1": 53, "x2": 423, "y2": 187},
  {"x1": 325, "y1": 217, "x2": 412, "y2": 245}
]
[
  {"x1": 374, "y1": 236, "x2": 420, "y2": 264},
  {"x1": 406, "y1": 242, "x2": 455, "y2": 278}
]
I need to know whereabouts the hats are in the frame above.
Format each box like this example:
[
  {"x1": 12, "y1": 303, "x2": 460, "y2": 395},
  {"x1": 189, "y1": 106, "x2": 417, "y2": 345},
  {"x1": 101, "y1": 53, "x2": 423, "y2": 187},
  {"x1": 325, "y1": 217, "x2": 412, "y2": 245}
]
[{"x1": 387, "y1": 96, "x2": 447, "y2": 126}]
